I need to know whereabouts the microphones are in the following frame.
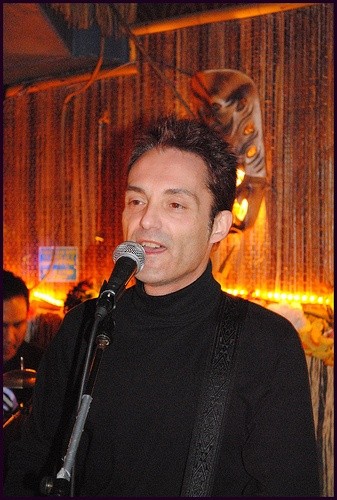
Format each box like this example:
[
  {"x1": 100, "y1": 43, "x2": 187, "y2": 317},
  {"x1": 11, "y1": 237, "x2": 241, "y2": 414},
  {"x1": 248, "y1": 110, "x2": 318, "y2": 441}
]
[{"x1": 93, "y1": 241, "x2": 146, "y2": 321}]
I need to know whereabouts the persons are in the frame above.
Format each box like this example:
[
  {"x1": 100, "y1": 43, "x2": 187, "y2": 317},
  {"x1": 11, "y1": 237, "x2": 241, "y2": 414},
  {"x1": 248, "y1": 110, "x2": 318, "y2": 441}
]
[
  {"x1": 4, "y1": 117, "x2": 319, "y2": 500},
  {"x1": 2, "y1": 270, "x2": 47, "y2": 499}
]
